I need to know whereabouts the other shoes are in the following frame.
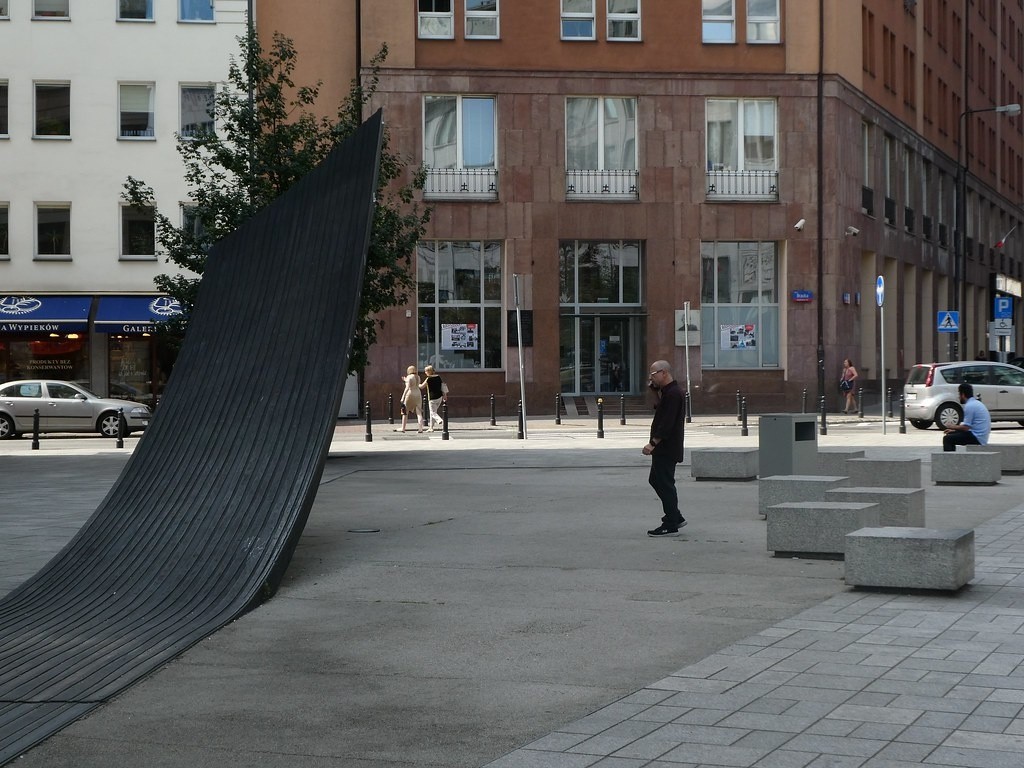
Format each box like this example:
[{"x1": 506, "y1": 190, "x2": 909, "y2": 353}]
[
  {"x1": 418, "y1": 431, "x2": 423, "y2": 433},
  {"x1": 851, "y1": 411, "x2": 859, "y2": 414},
  {"x1": 841, "y1": 410, "x2": 848, "y2": 415},
  {"x1": 427, "y1": 429, "x2": 434, "y2": 432},
  {"x1": 401, "y1": 431, "x2": 405, "y2": 433}
]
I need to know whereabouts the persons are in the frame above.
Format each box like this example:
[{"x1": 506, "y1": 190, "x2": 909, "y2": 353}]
[
  {"x1": 400, "y1": 366, "x2": 424, "y2": 434},
  {"x1": 943, "y1": 384, "x2": 991, "y2": 452},
  {"x1": 418, "y1": 365, "x2": 447, "y2": 432},
  {"x1": 679, "y1": 314, "x2": 697, "y2": 331},
  {"x1": 641, "y1": 360, "x2": 688, "y2": 537},
  {"x1": 976, "y1": 351, "x2": 987, "y2": 361},
  {"x1": 840, "y1": 358, "x2": 858, "y2": 414}
]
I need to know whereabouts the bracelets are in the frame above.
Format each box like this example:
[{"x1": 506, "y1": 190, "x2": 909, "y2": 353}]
[{"x1": 649, "y1": 440, "x2": 656, "y2": 447}]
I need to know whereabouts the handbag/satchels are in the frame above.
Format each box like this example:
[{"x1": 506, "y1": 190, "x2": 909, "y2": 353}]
[
  {"x1": 840, "y1": 380, "x2": 853, "y2": 391},
  {"x1": 400, "y1": 403, "x2": 407, "y2": 416},
  {"x1": 438, "y1": 374, "x2": 449, "y2": 393}
]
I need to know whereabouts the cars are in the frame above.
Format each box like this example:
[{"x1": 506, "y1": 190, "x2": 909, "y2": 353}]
[
  {"x1": 0, "y1": 380, "x2": 152, "y2": 438},
  {"x1": 61, "y1": 380, "x2": 155, "y2": 409},
  {"x1": 904, "y1": 362, "x2": 1024, "y2": 431}
]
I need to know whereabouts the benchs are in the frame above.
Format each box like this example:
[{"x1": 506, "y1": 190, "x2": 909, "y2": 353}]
[
  {"x1": 691, "y1": 448, "x2": 761, "y2": 482},
  {"x1": 757, "y1": 475, "x2": 852, "y2": 518},
  {"x1": 825, "y1": 487, "x2": 926, "y2": 528},
  {"x1": 844, "y1": 527, "x2": 976, "y2": 596},
  {"x1": 846, "y1": 457, "x2": 922, "y2": 490},
  {"x1": 966, "y1": 443, "x2": 1024, "y2": 471},
  {"x1": 818, "y1": 447, "x2": 866, "y2": 476},
  {"x1": 931, "y1": 451, "x2": 1002, "y2": 486},
  {"x1": 767, "y1": 502, "x2": 880, "y2": 560}
]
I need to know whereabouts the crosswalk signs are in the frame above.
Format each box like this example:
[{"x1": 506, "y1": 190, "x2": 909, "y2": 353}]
[{"x1": 938, "y1": 310, "x2": 959, "y2": 332}]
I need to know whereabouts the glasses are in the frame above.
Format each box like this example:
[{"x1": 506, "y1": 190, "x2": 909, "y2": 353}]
[{"x1": 649, "y1": 369, "x2": 664, "y2": 377}]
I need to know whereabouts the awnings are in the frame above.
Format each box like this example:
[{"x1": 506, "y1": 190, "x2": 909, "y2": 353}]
[
  {"x1": 93, "y1": 296, "x2": 191, "y2": 333},
  {"x1": 0, "y1": 297, "x2": 93, "y2": 333}
]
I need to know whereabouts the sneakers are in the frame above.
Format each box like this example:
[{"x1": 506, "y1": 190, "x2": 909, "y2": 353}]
[{"x1": 647, "y1": 516, "x2": 688, "y2": 537}]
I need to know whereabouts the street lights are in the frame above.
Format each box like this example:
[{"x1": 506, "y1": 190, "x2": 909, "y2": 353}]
[{"x1": 952, "y1": 104, "x2": 1022, "y2": 361}]
